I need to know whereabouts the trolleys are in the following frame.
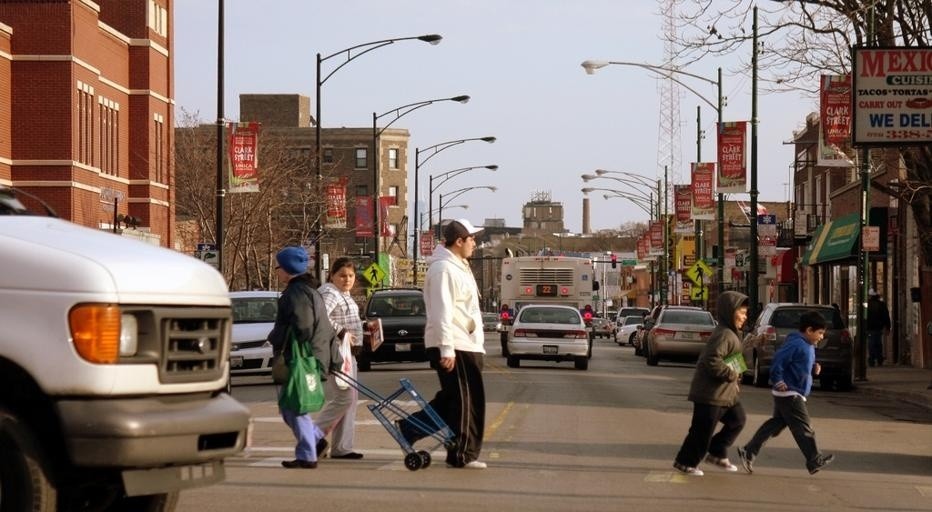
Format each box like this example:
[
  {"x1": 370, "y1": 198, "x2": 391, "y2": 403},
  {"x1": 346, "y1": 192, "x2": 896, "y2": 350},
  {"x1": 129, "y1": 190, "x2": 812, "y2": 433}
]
[{"x1": 328, "y1": 370, "x2": 459, "y2": 471}]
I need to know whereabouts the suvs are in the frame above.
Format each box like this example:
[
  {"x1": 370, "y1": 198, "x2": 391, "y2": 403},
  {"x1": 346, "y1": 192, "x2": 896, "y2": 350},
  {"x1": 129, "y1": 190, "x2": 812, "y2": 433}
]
[
  {"x1": 742, "y1": 303, "x2": 852, "y2": 393},
  {"x1": 1, "y1": 183, "x2": 255, "y2": 512}
]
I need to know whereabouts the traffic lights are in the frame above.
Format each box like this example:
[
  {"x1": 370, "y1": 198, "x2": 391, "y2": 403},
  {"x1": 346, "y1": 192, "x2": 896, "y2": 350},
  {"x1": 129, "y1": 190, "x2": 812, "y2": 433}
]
[{"x1": 611, "y1": 255, "x2": 617, "y2": 269}]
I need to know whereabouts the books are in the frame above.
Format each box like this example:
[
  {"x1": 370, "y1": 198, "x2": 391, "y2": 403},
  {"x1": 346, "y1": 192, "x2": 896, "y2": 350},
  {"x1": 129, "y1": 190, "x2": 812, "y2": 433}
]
[
  {"x1": 369, "y1": 318, "x2": 385, "y2": 352},
  {"x1": 723, "y1": 351, "x2": 749, "y2": 374}
]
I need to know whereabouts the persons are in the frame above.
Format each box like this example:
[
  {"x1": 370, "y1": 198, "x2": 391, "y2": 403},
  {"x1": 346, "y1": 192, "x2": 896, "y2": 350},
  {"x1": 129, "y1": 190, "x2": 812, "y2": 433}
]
[
  {"x1": 262, "y1": 245, "x2": 345, "y2": 470},
  {"x1": 312, "y1": 257, "x2": 375, "y2": 461},
  {"x1": 736, "y1": 311, "x2": 836, "y2": 476},
  {"x1": 832, "y1": 302, "x2": 846, "y2": 329},
  {"x1": 392, "y1": 217, "x2": 489, "y2": 471},
  {"x1": 672, "y1": 289, "x2": 751, "y2": 477},
  {"x1": 868, "y1": 286, "x2": 895, "y2": 367}
]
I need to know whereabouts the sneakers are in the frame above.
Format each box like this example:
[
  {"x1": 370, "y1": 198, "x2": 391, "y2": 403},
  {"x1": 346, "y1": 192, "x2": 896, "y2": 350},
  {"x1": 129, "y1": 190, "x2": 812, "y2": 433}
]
[
  {"x1": 673, "y1": 460, "x2": 704, "y2": 477},
  {"x1": 390, "y1": 419, "x2": 414, "y2": 457},
  {"x1": 330, "y1": 452, "x2": 363, "y2": 460},
  {"x1": 738, "y1": 446, "x2": 756, "y2": 475},
  {"x1": 446, "y1": 460, "x2": 487, "y2": 469},
  {"x1": 316, "y1": 437, "x2": 329, "y2": 459},
  {"x1": 806, "y1": 454, "x2": 836, "y2": 475},
  {"x1": 281, "y1": 459, "x2": 317, "y2": 468},
  {"x1": 703, "y1": 454, "x2": 737, "y2": 472}
]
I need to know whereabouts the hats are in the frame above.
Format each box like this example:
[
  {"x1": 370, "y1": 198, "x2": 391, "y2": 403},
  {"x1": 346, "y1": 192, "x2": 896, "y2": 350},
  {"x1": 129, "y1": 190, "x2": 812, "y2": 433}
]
[
  {"x1": 276, "y1": 246, "x2": 308, "y2": 275},
  {"x1": 444, "y1": 219, "x2": 484, "y2": 241}
]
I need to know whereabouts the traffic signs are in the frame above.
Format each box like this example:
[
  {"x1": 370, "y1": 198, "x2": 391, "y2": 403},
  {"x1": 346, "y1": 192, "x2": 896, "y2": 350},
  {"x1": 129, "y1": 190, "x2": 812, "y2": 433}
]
[{"x1": 622, "y1": 259, "x2": 637, "y2": 266}]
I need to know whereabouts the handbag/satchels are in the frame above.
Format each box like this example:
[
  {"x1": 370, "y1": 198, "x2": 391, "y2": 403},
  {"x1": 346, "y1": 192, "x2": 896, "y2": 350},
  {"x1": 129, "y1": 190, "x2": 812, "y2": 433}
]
[{"x1": 272, "y1": 348, "x2": 325, "y2": 416}]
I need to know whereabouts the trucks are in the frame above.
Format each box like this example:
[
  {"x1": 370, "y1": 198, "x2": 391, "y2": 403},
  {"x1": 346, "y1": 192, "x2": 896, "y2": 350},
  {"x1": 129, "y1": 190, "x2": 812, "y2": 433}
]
[{"x1": 500, "y1": 251, "x2": 596, "y2": 361}]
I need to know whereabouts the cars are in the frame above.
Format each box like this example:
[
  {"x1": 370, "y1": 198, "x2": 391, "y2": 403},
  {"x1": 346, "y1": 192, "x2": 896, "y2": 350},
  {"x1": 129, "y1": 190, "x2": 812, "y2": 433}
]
[
  {"x1": 483, "y1": 314, "x2": 501, "y2": 333},
  {"x1": 594, "y1": 302, "x2": 719, "y2": 369},
  {"x1": 351, "y1": 290, "x2": 438, "y2": 372},
  {"x1": 507, "y1": 303, "x2": 591, "y2": 372},
  {"x1": 227, "y1": 287, "x2": 284, "y2": 380}
]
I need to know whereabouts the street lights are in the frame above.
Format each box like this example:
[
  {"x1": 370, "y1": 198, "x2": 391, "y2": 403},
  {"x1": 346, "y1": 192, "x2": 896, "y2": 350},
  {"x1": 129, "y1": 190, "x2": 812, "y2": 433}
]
[
  {"x1": 580, "y1": 163, "x2": 662, "y2": 233},
  {"x1": 418, "y1": 205, "x2": 468, "y2": 264},
  {"x1": 371, "y1": 89, "x2": 469, "y2": 290},
  {"x1": 430, "y1": 162, "x2": 499, "y2": 253},
  {"x1": 438, "y1": 185, "x2": 499, "y2": 248},
  {"x1": 311, "y1": 33, "x2": 446, "y2": 284},
  {"x1": 410, "y1": 133, "x2": 500, "y2": 290},
  {"x1": 581, "y1": 57, "x2": 725, "y2": 292}
]
